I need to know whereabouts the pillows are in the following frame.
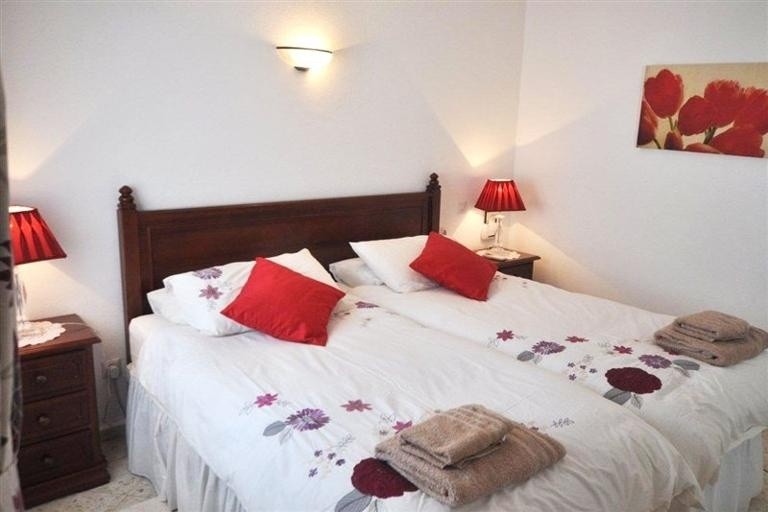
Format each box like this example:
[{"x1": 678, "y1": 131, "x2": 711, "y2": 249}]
[
  {"x1": 409, "y1": 230, "x2": 497, "y2": 302},
  {"x1": 144, "y1": 285, "x2": 213, "y2": 330},
  {"x1": 349, "y1": 235, "x2": 443, "y2": 293},
  {"x1": 162, "y1": 245, "x2": 355, "y2": 334},
  {"x1": 328, "y1": 255, "x2": 384, "y2": 286},
  {"x1": 218, "y1": 257, "x2": 348, "y2": 347}
]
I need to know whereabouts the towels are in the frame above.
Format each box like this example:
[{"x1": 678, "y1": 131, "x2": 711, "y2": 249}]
[
  {"x1": 673, "y1": 309, "x2": 751, "y2": 342},
  {"x1": 653, "y1": 318, "x2": 767, "y2": 367},
  {"x1": 374, "y1": 419, "x2": 567, "y2": 508},
  {"x1": 396, "y1": 403, "x2": 514, "y2": 471}
]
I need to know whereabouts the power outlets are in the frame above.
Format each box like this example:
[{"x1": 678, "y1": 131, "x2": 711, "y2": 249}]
[{"x1": 101, "y1": 358, "x2": 123, "y2": 382}]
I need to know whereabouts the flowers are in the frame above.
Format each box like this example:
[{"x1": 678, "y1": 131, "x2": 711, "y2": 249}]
[{"x1": 636, "y1": 69, "x2": 768, "y2": 158}]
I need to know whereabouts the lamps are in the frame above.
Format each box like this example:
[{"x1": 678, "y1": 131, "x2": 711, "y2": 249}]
[
  {"x1": 8, "y1": 205, "x2": 68, "y2": 336},
  {"x1": 276, "y1": 45, "x2": 335, "y2": 72},
  {"x1": 473, "y1": 178, "x2": 528, "y2": 250}
]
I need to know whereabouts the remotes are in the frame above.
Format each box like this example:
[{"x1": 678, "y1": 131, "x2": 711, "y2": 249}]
[{"x1": 483, "y1": 251, "x2": 505, "y2": 262}]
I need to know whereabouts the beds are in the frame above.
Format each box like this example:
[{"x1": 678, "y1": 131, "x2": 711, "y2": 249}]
[{"x1": 116, "y1": 172, "x2": 768, "y2": 512}]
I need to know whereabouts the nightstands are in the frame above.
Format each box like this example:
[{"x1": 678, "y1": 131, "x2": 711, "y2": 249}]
[
  {"x1": 12, "y1": 313, "x2": 112, "y2": 511},
  {"x1": 471, "y1": 245, "x2": 542, "y2": 280}
]
